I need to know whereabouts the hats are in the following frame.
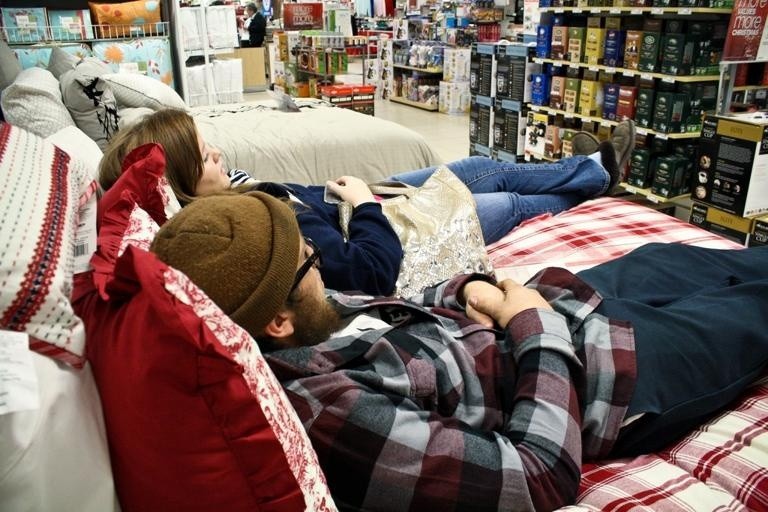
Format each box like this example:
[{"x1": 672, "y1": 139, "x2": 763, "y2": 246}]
[{"x1": 149, "y1": 190, "x2": 300, "y2": 338}]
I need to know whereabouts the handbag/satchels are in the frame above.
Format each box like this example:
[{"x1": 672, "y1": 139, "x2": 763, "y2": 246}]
[{"x1": 337, "y1": 169, "x2": 496, "y2": 300}]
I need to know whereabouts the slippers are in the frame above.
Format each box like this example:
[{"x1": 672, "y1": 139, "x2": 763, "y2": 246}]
[
  {"x1": 571, "y1": 132, "x2": 599, "y2": 156},
  {"x1": 594, "y1": 119, "x2": 636, "y2": 196}
]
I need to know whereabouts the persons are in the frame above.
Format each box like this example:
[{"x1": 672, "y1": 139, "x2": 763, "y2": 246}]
[
  {"x1": 239, "y1": 2, "x2": 266, "y2": 49},
  {"x1": 95, "y1": 105, "x2": 637, "y2": 298},
  {"x1": 147, "y1": 190, "x2": 768, "y2": 512}
]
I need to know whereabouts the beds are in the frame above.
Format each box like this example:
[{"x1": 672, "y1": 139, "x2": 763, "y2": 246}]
[{"x1": 484, "y1": 194, "x2": 768, "y2": 511}]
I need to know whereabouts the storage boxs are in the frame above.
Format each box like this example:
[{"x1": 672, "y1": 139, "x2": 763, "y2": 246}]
[{"x1": 688, "y1": 108, "x2": 768, "y2": 247}]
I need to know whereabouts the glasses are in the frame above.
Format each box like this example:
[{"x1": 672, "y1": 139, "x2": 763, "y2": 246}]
[{"x1": 289, "y1": 235, "x2": 324, "y2": 294}]
